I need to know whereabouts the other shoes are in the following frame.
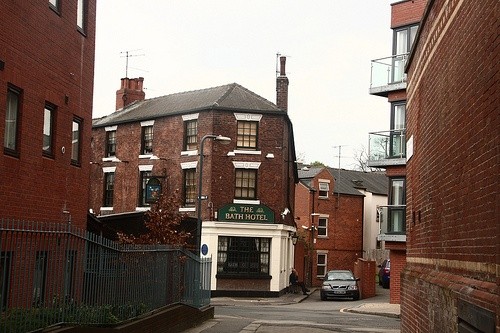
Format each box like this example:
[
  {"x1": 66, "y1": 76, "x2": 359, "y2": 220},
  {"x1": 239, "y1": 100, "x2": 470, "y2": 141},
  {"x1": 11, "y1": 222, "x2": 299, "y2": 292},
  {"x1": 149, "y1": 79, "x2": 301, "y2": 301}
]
[
  {"x1": 303, "y1": 293, "x2": 308, "y2": 295},
  {"x1": 304, "y1": 289, "x2": 310, "y2": 292}
]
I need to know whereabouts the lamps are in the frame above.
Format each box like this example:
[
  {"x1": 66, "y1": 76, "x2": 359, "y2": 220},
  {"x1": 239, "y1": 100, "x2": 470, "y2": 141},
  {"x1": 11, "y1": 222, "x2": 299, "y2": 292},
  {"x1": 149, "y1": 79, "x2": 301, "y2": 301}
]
[
  {"x1": 112, "y1": 158, "x2": 129, "y2": 163},
  {"x1": 266, "y1": 154, "x2": 275, "y2": 158},
  {"x1": 289, "y1": 232, "x2": 300, "y2": 246},
  {"x1": 281, "y1": 207, "x2": 290, "y2": 218},
  {"x1": 149, "y1": 156, "x2": 168, "y2": 163},
  {"x1": 227, "y1": 152, "x2": 235, "y2": 157}
]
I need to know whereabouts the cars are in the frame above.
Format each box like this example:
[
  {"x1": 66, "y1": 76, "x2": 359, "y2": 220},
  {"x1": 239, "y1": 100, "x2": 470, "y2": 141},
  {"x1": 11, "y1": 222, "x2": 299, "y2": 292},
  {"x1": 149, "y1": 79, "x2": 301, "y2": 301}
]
[
  {"x1": 318, "y1": 270, "x2": 361, "y2": 300},
  {"x1": 377, "y1": 259, "x2": 391, "y2": 289}
]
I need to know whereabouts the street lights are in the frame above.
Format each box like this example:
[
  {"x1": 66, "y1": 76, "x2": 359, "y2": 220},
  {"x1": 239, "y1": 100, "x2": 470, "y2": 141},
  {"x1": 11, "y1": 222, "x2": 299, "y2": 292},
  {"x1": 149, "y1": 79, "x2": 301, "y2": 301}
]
[{"x1": 197, "y1": 135, "x2": 231, "y2": 256}]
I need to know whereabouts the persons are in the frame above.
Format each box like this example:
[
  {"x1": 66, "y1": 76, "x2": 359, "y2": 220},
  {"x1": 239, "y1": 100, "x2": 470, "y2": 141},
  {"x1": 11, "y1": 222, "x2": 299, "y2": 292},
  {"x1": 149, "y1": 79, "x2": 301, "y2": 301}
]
[{"x1": 289, "y1": 267, "x2": 312, "y2": 298}]
